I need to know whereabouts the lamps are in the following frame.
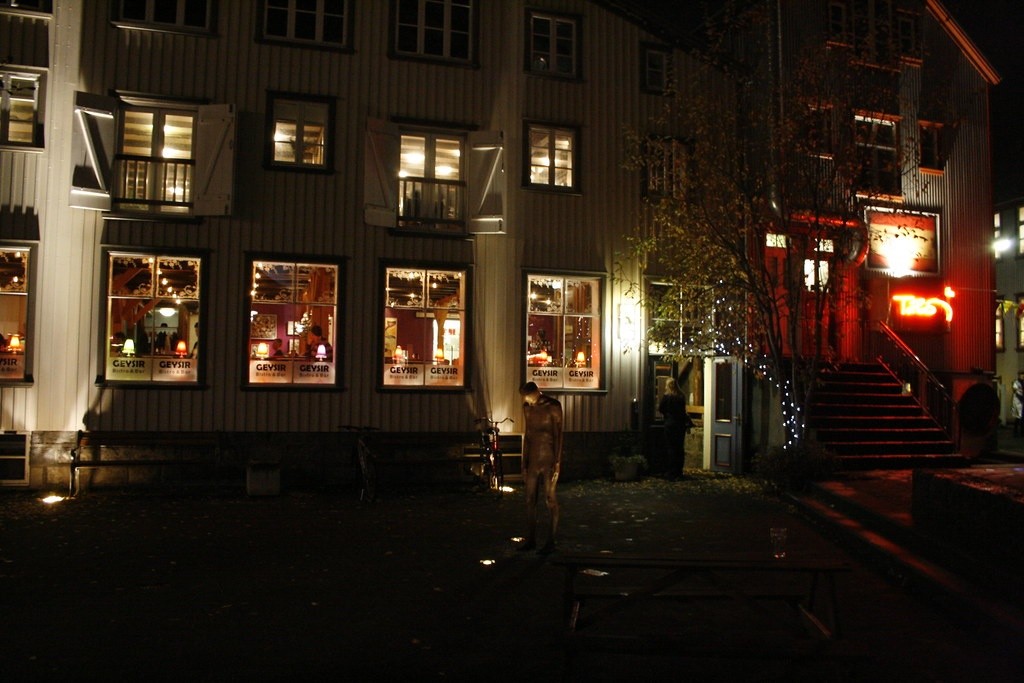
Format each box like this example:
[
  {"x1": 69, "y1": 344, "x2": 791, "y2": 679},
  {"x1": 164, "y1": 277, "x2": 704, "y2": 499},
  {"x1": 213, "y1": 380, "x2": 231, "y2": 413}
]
[
  {"x1": 257, "y1": 342, "x2": 268, "y2": 361},
  {"x1": 175, "y1": 341, "x2": 188, "y2": 359},
  {"x1": 122, "y1": 339, "x2": 136, "y2": 357},
  {"x1": 7, "y1": 334, "x2": 22, "y2": 354},
  {"x1": 316, "y1": 344, "x2": 328, "y2": 362}
]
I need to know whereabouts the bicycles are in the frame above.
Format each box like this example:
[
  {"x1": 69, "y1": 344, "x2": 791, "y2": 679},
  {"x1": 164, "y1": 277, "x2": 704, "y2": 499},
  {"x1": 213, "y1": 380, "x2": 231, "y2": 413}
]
[
  {"x1": 339, "y1": 424, "x2": 380, "y2": 509},
  {"x1": 474, "y1": 415, "x2": 515, "y2": 500}
]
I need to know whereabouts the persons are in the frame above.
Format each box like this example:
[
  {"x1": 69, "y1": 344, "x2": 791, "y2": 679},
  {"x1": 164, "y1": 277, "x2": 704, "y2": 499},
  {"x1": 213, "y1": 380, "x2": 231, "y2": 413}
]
[
  {"x1": 1011, "y1": 370, "x2": 1024, "y2": 438},
  {"x1": 516, "y1": 383, "x2": 563, "y2": 557},
  {"x1": 189, "y1": 322, "x2": 199, "y2": 361},
  {"x1": 827, "y1": 313, "x2": 838, "y2": 363},
  {"x1": 309, "y1": 325, "x2": 333, "y2": 361},
  {"x1": 156, "y1": 324, "x2": 172, "y2": 356},
  {"x1": 815, "y1": 311, "x2": 823, "y2": 358}
]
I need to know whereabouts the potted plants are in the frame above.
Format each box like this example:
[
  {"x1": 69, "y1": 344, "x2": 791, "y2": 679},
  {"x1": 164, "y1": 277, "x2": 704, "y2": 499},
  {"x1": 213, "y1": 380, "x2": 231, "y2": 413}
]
[{"x1": 610, "y1": 427, "x2": 647, "y2": 481}]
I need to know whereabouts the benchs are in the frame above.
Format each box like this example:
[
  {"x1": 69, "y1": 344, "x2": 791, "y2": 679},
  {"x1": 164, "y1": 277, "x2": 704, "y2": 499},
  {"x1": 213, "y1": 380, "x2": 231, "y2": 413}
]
[
  {"x1": 68, "y1": 430, "x2": 223, "y2": 499},
  {"x1": 556, "y1": 552, "x2": 846, "y2": 640}
]
